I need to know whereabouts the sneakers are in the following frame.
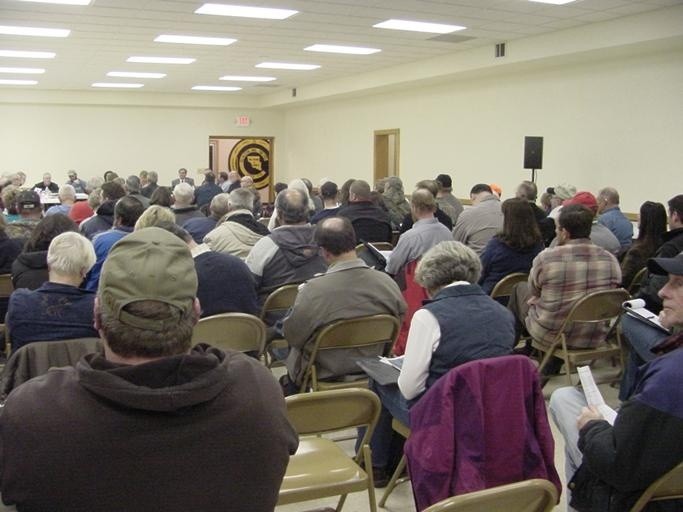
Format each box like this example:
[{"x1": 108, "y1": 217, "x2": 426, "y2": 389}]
[
  {"x1": 280, "y1": 376, "x2": 296, "y2": 395},
  {"x1": 391, "y1": 462, "x2": 408, "y2": 478},
  {"x1": 352, "y1": 456, "x2": 390, "y2": 488}
]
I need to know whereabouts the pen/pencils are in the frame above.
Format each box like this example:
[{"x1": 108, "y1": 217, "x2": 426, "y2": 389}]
[{"x1": 648, "y1": 316, "x2": 654, "y2": 319}]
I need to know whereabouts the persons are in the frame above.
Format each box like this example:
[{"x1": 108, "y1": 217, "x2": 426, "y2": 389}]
[{"x1": 0, "y1": 167, "x2": 682, "y2": 512}]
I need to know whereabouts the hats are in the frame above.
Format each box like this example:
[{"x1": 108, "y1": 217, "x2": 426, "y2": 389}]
[
  {"x1": 490, "y1": 184, "x2": 501, "y2": 194},
  {"x1": 67, "y1": 170, "x2": 76, "y2": 176},
  {"x1": 562, "y1": 192, "x2": 599, "y2": 209},
  {"x1": 321, "y1": 182, "x2": 342, "y2": 196},
  {"x1": 99, "y1": 227, "x2": 198, "y2": 331},
  {"x1": 547, "y1": 185, "x2": 576, "y2": 200},
  {"x1": 436, "y1": 175, "x2": 451, "y2": 188},
  {"x1": 17, "y1": 190, "x2": 44, "y2": 209},
  {"x1": 647, "y1": 252, "x2": 683, "y2": 276}
]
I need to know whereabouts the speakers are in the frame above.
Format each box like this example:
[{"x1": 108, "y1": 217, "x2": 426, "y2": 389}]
[{"x1": 524, "y1": 136, "x2": 543, "y2": 169}]
